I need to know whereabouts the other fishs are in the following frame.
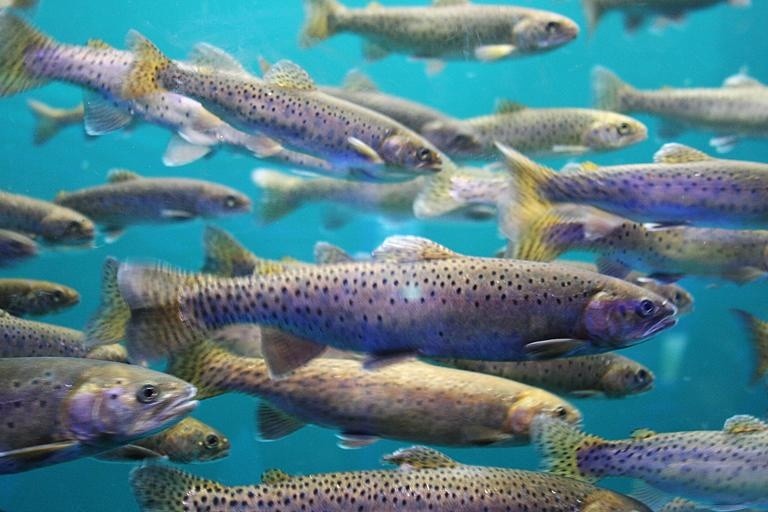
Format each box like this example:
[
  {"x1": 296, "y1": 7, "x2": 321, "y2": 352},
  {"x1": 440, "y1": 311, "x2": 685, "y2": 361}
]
[
  {"x1": 297, "y1": 2, "x2": 583, "y2": 67},
  {"x1": 584, "y1": 2, "x2": 739, "y2": 33},
  {"x1": 0, "y1": 18, "x2": 767, "y2": 511}
]
